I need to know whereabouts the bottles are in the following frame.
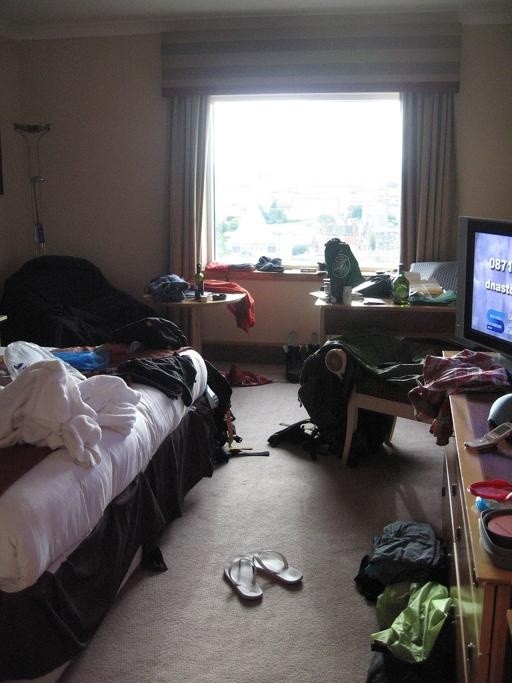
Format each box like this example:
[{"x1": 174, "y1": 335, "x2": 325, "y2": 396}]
[
  {"x1": 392, "y1": 264, "x2": 410, "y2": 305},
  {"x1": 194, "y1": 263, "x2": 205, "y2": 300}
]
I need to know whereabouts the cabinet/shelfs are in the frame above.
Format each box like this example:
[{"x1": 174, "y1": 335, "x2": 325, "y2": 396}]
[{"x1": 438, "y1": 394, "x2": 511, "y2": 683}]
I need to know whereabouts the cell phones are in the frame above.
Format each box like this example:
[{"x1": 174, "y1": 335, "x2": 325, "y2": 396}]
[{"x1": 464, "y1": 422, "x2": 512, "y2": 451}]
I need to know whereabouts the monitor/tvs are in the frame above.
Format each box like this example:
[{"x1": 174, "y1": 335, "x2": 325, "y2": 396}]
[{"x1": 455, "y1": 216, "x2": 512, "y2": 359}]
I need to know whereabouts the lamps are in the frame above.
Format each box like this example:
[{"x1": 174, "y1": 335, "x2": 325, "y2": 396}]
[{"x1": 10, "y1": 121, "x2": 53, "y2": 255}]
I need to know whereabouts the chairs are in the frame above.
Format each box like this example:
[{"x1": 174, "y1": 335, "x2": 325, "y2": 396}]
[
  {"x1": 8, "y1": 253, "x2": 166, "y2": 346},
  {"x1": 319, "y1": 329, "x2": 455, "y2": 469}
]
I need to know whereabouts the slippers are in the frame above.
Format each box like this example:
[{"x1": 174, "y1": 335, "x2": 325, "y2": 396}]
[{"x1": 225, "y1": 549, "x2": 304, "y2": 601}]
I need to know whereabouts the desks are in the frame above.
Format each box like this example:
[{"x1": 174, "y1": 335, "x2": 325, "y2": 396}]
[
  {"x1": 315, "y1": 296, "x2": 456, "y2": 351},
  {"x1": 142, "y1": 290, "x2": 247, "y2": 357}
]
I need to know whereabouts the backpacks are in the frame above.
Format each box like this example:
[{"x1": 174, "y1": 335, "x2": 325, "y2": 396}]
[{"x1": 299, "y1": 339, "x2": 390, "y2": 459}]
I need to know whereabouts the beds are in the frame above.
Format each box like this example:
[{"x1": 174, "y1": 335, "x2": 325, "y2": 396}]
[{"x1": 2, "y1": 343, "x2": 218, "y2": 681}]
